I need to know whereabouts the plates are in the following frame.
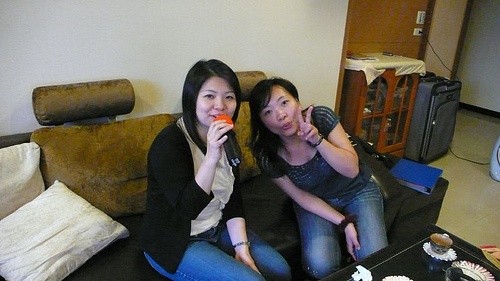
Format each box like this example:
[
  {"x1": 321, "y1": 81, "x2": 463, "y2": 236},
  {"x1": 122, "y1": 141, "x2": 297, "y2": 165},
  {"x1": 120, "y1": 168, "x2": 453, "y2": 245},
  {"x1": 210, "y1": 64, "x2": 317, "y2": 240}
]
[
  {"x1": 422, "y1": 242, "x2": 457, "y2": 261},
  {"x1": 381, "y1": 276, "x2": 414, "y2": 281},
  {"x1": 452, "y1": 259, "x2": 495, "y2": 281}
]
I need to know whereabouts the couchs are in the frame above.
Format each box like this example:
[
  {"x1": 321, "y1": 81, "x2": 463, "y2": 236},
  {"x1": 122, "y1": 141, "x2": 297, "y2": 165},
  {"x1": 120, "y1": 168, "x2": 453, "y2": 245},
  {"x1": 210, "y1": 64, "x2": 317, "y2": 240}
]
[{"x1": 0, "y1": 71, "x2": 401, "y2": 281}]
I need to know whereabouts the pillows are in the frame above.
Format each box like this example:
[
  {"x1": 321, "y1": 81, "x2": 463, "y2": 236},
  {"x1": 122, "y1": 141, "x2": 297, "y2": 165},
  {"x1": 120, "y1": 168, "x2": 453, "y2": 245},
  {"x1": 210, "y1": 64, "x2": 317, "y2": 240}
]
[
  {"x1": 30, "y1": 114, "x2": 173, "y2": 218},
  {"x1": 0, "y1": 142, "x2": 46, "y2": 221},
  {"x1": 0, "y1": 181, "x2": 129, "y2": 281}
]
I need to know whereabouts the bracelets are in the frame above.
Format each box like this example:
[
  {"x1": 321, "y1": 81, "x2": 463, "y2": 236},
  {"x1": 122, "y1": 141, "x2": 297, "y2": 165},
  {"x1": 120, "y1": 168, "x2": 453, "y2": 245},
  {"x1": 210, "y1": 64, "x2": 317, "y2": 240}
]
[
  {"x1": 233, "y1": 241, "x2": 250, "y2": 249},
  {"x1": 338, "y1": 215, "x2": 353, "y2": 231},
  {"x1": 307, "y1": 133, "x2": 323, "y2": 147}
]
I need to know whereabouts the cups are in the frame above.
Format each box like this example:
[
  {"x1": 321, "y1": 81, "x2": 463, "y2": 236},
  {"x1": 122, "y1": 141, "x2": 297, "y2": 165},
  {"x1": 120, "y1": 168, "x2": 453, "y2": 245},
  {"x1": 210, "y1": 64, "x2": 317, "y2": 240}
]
[
  {"x1": 430, "y1": 233, "x2": 453, "y2": 254},
  {"x1": 445, "y1": 267, "x2": 463, "y2": 281}
]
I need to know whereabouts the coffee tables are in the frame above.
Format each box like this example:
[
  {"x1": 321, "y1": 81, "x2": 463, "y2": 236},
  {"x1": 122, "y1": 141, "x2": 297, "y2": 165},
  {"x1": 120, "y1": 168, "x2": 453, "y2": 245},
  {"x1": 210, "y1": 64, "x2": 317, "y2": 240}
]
[{"x1": 323, "y1": 223, "x2": 500, "y2": 281}]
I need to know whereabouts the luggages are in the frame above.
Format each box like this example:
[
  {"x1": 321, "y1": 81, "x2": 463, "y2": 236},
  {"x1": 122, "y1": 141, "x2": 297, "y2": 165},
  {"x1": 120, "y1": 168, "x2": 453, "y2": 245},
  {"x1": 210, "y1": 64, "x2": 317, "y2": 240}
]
[{"x1": 404, "y1": 71, "x2": 462, "y2": 163}]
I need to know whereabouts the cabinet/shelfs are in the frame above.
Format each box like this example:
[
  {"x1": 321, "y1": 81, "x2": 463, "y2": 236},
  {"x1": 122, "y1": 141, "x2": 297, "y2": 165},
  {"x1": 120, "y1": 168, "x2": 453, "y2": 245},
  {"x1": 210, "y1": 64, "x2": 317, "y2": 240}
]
[{"x1": 342, "y1": 52, "x2": 423, "y2": 155}]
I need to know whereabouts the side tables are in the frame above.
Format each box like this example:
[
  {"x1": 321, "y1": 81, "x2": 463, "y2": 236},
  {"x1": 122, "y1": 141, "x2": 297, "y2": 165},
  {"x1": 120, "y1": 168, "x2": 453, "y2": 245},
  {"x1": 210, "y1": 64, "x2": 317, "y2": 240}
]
[{"x1": 387, "y1": 153, "x2": 448, "y2": 246}]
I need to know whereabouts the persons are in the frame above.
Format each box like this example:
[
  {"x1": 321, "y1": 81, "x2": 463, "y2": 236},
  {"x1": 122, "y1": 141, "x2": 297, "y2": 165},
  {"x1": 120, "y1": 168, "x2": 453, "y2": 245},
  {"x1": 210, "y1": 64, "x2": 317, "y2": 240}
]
[
  {"x1": 244, "y1": 77, "x2": 389, "y2": 281},
  {"x1": 141, "y1": 60, "x2": 291, "y2": 281}
]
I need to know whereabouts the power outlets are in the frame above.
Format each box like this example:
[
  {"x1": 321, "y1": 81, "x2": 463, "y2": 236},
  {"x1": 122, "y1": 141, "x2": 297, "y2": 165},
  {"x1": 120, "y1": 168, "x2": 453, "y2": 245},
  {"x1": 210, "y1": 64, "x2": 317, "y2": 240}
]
[{"x1": 414, "y1": 28, "x2": 423, "y2": 36}]
